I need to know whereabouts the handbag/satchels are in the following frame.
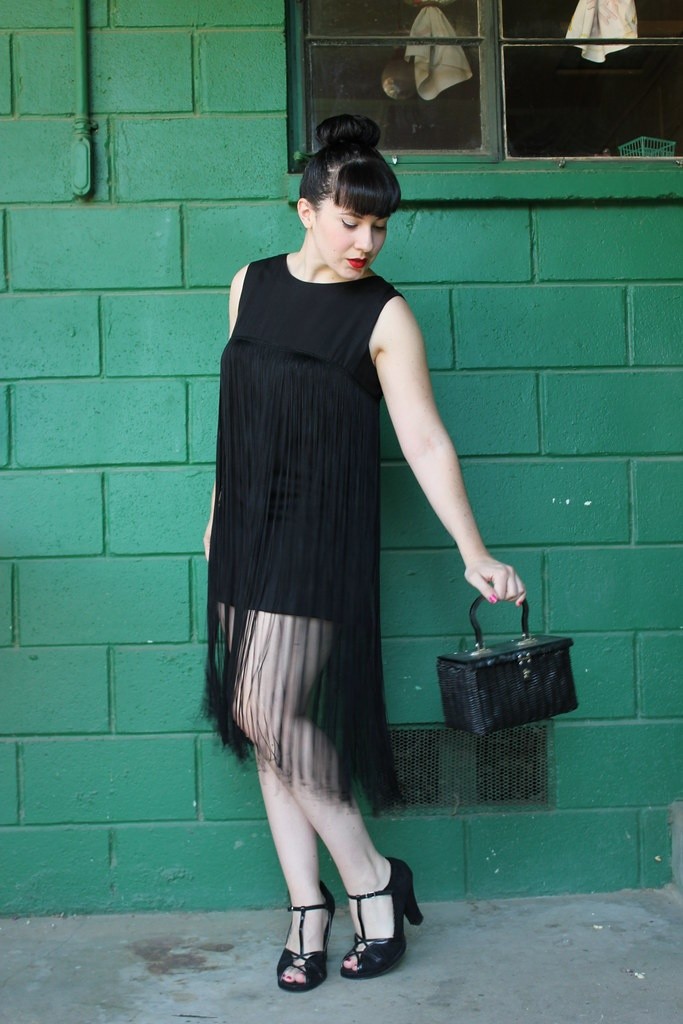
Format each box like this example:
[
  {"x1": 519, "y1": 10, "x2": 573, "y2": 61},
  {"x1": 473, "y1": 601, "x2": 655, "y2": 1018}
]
[{"x1": 437, "y1": 593, "x2": 578, "y2": 736}]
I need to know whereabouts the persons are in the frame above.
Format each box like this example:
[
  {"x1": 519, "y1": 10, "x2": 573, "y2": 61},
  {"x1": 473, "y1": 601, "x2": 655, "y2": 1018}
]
[{"x1": 205, "y1": 113, "x2": 527, "y2": 993}]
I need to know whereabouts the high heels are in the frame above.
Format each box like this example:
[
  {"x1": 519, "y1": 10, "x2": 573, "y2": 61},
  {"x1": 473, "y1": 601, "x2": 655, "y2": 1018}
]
[
  {"x1": 277, "y1": 879, "x2": 335, "y2": 991},
  {"x1": 341, "y1": 857, "x2": 424, "y2": 978}
]
